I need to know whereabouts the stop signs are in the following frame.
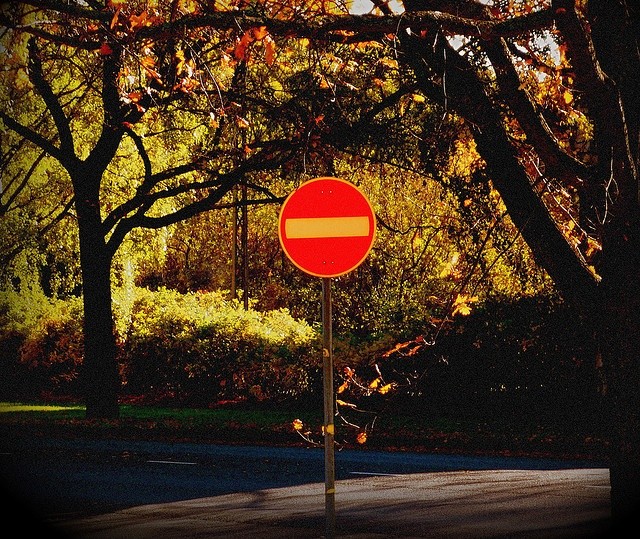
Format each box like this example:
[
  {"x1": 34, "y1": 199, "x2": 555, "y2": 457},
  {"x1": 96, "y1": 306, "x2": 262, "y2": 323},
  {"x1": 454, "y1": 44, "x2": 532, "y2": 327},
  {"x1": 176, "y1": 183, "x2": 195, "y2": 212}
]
[{"x1": 277, "y1": 177, "x2": 377, "y2": 278}]
